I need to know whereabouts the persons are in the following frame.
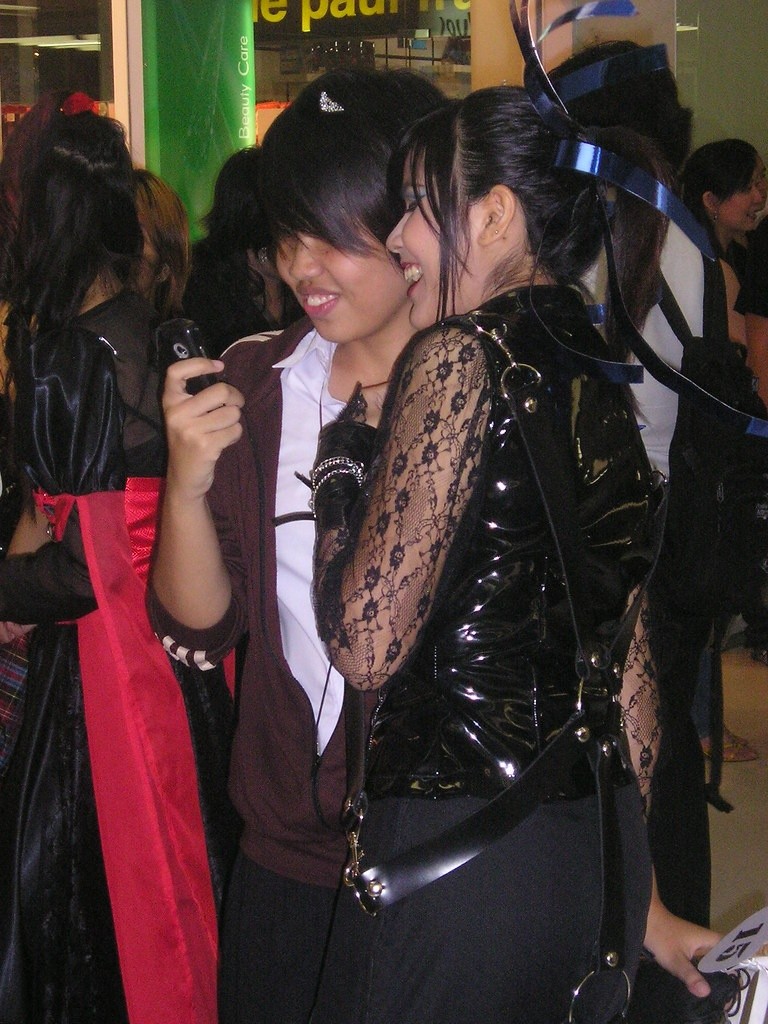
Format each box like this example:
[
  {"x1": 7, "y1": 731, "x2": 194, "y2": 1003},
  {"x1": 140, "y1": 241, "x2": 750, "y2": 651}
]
[{"x1": 0, "y1": 41, "x2": 768, "y2": 1024}]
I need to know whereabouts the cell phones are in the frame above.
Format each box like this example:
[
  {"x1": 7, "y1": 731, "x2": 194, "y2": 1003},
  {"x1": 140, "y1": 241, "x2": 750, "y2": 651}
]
[{"x1": 162, "y1": 318, "x2": 216, "y2": 395}]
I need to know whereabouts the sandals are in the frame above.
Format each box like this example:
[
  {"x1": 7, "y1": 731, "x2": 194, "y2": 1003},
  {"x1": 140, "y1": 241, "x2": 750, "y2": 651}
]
[{"x1": 700, "y1": 723, "x2": 759, "y2": 762}]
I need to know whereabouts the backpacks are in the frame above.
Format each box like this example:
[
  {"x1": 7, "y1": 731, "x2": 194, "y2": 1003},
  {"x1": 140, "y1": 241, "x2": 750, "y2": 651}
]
[{"x1": 649, "y1": 252, "x2": 768, "y2": 620}]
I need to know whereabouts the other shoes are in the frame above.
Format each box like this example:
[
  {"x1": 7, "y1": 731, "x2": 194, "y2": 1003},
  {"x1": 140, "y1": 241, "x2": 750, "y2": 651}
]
[{"x1": 749, "y1": 648, "x2": 767, "y2": 666}]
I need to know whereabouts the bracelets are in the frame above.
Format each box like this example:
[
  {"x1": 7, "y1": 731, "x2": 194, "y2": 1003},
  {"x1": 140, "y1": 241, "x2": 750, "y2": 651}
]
[{"x1": 307, "y1": 455, "x2": 365, "y2": 519}]
